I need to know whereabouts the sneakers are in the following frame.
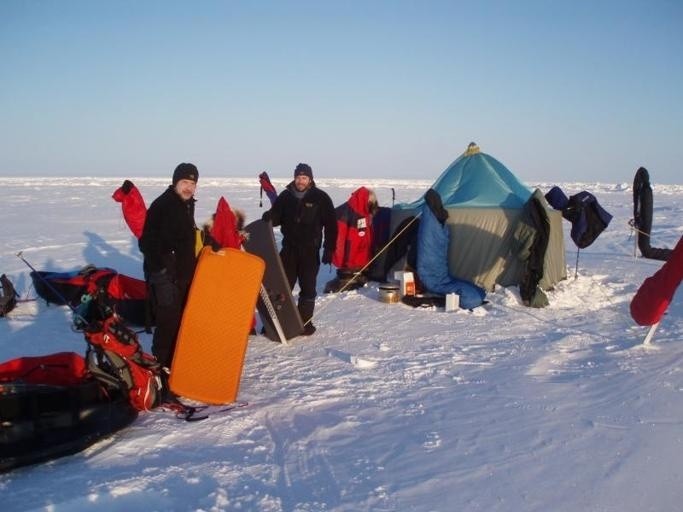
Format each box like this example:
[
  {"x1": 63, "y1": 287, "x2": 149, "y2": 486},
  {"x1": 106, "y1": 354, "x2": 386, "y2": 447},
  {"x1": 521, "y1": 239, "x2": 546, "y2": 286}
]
[{"x1": 303, "y1": 323, "x2": 315, "y2": 334}]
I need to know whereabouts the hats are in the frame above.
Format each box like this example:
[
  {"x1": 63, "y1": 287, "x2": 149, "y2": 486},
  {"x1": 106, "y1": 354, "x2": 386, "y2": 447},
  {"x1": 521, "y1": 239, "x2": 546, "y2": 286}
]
[
  {"x1": 172, "y1": 162, "x2": 198, "y2": 187},
  {"x1": 295, "y1": 164, "x2": 314, "y2": 177}
]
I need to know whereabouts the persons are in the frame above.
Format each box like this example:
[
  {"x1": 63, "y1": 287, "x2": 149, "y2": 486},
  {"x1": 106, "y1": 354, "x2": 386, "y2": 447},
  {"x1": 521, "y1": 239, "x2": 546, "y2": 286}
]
[
  {"x1": 262, "y1": 161, "x2": 337, "y2": 330},
  {"x1": 142, "y1": 162, "x2": 199, "y2": 367}
]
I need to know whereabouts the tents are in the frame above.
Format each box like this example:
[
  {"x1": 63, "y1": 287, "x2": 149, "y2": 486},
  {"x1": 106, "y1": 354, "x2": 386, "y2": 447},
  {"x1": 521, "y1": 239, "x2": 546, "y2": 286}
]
[{"x1": 389, "y1": 141, "x2": 567, "y2": 293}]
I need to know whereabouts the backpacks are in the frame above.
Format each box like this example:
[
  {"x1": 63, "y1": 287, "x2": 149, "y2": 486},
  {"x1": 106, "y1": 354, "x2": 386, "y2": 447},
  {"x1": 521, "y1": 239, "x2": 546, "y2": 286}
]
[{"x1": 71, "y1": 300, "x2": 164, "y2": 411}]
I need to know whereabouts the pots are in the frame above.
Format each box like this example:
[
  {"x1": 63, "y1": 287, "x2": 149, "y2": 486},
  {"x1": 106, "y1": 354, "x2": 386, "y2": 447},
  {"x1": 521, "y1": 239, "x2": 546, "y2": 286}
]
[{"x1": 379, "y1": 285, "x2": 400, "y2": 303}]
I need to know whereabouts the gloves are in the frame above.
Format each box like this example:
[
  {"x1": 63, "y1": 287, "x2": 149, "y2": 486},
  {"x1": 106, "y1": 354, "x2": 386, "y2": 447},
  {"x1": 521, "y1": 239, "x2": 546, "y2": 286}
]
[
  {"x1": 261, "y1": 211, "x2": 272, "y2": 223},
  {"x1": 322, "y1": 249, "x2": 332, "y2": 266}
]
